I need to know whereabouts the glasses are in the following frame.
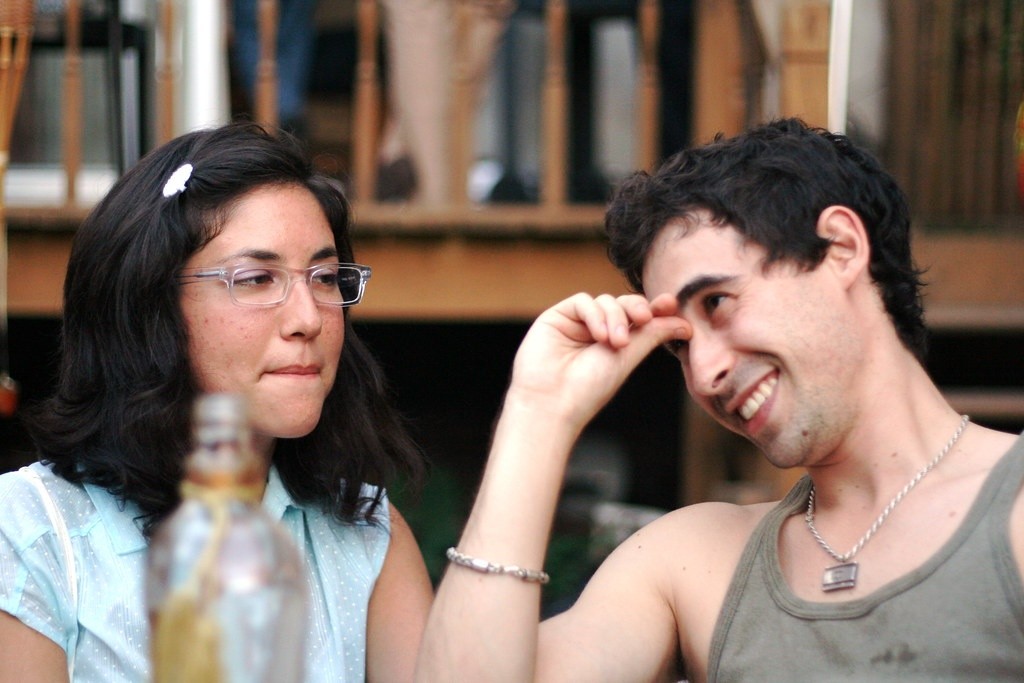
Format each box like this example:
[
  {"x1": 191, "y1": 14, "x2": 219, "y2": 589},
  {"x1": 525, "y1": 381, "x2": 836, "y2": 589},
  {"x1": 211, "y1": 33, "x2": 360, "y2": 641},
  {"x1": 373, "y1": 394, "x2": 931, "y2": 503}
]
[{"x1": 171, "y1": 262, "x2": 372, "y2": 306}]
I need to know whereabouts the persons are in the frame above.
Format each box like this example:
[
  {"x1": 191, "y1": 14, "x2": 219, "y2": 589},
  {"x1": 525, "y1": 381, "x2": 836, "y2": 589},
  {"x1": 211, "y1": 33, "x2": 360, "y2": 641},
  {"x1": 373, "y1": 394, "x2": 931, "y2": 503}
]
[
  {"x1": 416, "y1": 120, "x2": 1024, "y2": 683},
  {"x1": 0, "y1": 121, "x2": 437, "y2": 683}
]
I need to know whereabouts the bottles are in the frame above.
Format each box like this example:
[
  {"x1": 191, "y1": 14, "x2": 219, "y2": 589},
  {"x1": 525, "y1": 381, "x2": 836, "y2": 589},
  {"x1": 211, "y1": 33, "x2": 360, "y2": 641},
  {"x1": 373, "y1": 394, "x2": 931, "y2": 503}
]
[{"x1": 150, "y1": 394, "x2": 308, "y2": 683}]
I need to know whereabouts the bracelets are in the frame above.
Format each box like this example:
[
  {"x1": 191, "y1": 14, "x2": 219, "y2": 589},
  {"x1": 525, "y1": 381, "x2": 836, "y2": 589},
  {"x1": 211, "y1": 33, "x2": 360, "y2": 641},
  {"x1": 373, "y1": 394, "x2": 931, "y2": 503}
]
[{"x1": 445, "y1": 547, "x2": 549, "y2": 587}]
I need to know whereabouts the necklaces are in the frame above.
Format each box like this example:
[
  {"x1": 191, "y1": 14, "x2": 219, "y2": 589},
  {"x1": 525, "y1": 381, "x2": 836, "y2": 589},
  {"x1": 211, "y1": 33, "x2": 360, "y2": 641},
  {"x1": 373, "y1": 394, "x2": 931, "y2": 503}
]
[{"x1": 806, "y1": 414, "x2": 973, "y2": 593}]
